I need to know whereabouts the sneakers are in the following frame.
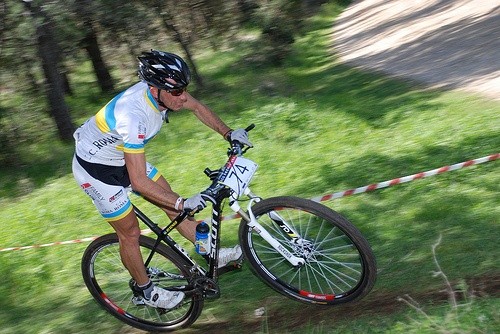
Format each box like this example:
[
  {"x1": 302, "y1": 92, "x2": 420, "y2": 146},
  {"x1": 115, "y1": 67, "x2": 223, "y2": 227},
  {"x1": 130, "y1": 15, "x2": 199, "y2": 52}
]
[
  {"x1": 207, "y1": 244, "x2": 244, "y2": 270},
  {"x1": 131, "y1": 285, "x2": 185, "y2": 309}
]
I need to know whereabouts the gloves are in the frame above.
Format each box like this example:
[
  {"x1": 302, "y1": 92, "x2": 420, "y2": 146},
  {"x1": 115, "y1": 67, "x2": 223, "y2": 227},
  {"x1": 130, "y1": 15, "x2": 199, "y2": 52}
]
[
  {"x1": 183, "y1": 193, "x2": 217, "y2": 212},
  {"x1": 224, "y1": 128, "x2": 254, "y2": 147}
]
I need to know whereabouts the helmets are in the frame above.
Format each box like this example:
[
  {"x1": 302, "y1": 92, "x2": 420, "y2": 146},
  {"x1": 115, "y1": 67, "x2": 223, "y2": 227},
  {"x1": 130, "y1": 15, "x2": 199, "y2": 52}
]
[{"x1": 137, "y1": 49, "x2": 191, "y2": 91}]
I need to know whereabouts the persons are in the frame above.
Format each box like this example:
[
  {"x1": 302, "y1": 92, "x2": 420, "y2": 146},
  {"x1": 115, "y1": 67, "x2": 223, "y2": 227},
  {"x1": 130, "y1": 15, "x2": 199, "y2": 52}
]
[{"x1": 71, "y1": 49, "x2": 254, "y2": 310}]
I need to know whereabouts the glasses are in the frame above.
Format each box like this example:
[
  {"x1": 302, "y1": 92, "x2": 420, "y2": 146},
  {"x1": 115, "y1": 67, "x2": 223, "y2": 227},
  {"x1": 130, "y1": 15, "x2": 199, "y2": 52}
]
[{"x1": 166, "y1": 87, "x2": 187, "y2": 96}]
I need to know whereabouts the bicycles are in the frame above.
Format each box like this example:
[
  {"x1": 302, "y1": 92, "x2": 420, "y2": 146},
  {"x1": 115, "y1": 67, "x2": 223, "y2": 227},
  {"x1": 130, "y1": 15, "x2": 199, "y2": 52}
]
[{"x1": 80, "y1": 122, "x2": 378, "y2": 333}]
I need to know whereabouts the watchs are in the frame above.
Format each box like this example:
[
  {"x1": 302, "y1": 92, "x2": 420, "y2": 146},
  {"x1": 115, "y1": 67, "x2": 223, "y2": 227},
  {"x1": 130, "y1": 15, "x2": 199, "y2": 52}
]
[{"x1": 175, "y1": 196, "x2": 183, "y2": 210}]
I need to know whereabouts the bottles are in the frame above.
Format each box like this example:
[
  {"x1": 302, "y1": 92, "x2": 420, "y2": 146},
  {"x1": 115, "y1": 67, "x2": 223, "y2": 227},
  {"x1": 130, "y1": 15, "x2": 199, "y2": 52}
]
[{"x1": 195, "y1": 222, "x2": 210, "y2": 255}]
